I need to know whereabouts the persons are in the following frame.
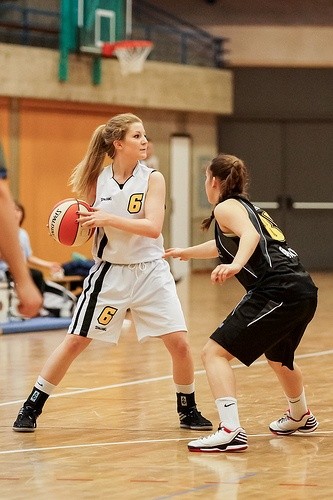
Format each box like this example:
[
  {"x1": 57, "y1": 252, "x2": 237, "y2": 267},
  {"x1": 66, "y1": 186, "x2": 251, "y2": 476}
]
[
  {"x1": 0, "y1": 141, "x2": 43, "y2": 315},
  {"x1": 14, "y1": 114, "x2": 214, "y2": 432},
  {"x1": 164, "y1": 154, "x2": 318, "y2": 453},
  {"x1": 12, "y1": 201, "x2": 64, "y2": 316},
  {"x1": 139, "y1": 138, "x2": 158, "y2": 172}
]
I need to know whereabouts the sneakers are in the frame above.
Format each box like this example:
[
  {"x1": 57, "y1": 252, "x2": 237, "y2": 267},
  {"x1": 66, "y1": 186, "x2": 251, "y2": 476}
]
[
  {"x1": 268, "y1": 408, "x2": 319, "y2": 436},
  {"x1": 179, "y1": 406, "x2": 213, "y2": 430},
  {"x1": 187, "y1": 421, "x2": 248, "y2": 452},
  {"x1": 13, "y1": 403, "x2": 42, "y2": 432}
]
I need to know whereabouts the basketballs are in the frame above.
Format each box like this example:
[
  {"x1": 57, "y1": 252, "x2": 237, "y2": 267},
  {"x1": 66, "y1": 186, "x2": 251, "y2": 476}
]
[{"x1": 48, "y1": 197, "x2": 97, "y2": 248}]
